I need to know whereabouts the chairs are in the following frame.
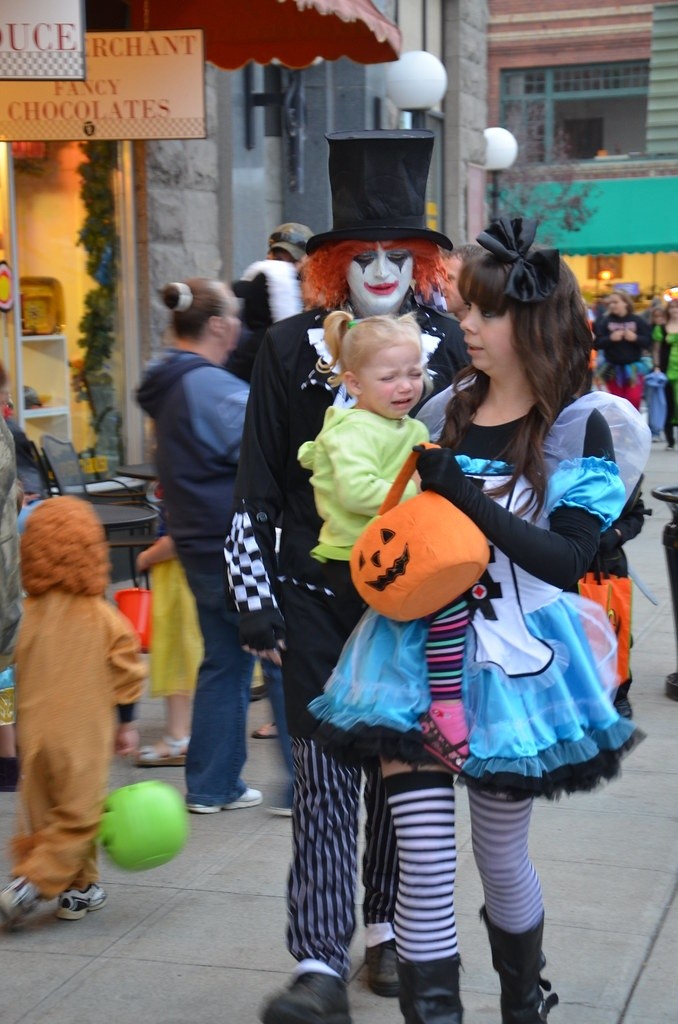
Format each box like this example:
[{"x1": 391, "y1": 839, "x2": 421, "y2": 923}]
[{"x1": 14, "y1": 435, "x2": 165, "y2": 590}]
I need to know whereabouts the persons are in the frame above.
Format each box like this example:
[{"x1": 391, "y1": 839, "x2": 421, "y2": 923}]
[
  {"x1": 297, "y1": 310, "x2": 472, "y2": 774},
  {"x1": 0, "y1": 495, "x2": 147, "y2": 931},
  {"x1": 591, "y1": 290, "x2": 678, "y2": 451},
  {"x1": 225, "y1": 224, "x2": 314, "y2": 741},
  {"x1": 139, "y1": 498, "x2": 205, "y2": 767},
  {"x1": 224, "y1": 128, "x2": 474, "y2": 1024},
  {"x1": 586, "y1": 497, "x2": 645, "y2": 722},
  {"x1": 298, "y1": 217, "x2": 652, "y2": 1024},
  {"x1": 134, "y1": 277, "x2": 294, "y2": 815}
]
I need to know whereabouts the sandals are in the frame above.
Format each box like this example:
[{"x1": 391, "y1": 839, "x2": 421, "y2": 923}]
[
  {"x1": 133, "y1": 735, "x2": 190, "y2": 766},
  {"x1": 252, "y1": 720, "x2": 278, "y2": 738}
]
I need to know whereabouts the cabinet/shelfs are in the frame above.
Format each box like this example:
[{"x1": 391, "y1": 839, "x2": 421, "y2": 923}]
[{"x1": 21, "y1": 330, "x2": 74, "y2": 448}]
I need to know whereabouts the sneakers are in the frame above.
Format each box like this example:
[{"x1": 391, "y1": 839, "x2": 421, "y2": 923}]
[
  {"x1": 264, "y1": 972, "x2": 351, "y2": 1024},
  {"x1": 187, "y1": 788, "x2": 263, "y2": 814},
  {"x1": 267, "y1": 796, "x2": 293, "y2": 816},
  {"x1": 56, "y1": 882, "x2": 107, "y2": 920},
  {"x1": 0, "y1": 876, "x2": 39, "y2": 928},
  {"x1": 348, "y1": 939, "x2": 400, "y2": 997}
]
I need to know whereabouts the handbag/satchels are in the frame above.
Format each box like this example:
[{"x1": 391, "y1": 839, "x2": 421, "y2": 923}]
[{"x1": 577, "y1": 543, "x2": 632, "y2": 684}]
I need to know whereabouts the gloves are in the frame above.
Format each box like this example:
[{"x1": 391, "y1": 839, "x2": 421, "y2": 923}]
[
  {"x1": 413, "y1": 445, "x2": 573, "y2": 588},
  {"x1": 598, "y1": 529, "x2": 625, "y2": 552}
]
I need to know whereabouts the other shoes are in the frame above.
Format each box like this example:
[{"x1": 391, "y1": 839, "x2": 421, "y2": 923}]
[
  {"x1": 0, "y1": 756, "x2": 21, "y2": 792},
  {"x1": 613, "y1": 698, "x2": 631, "y2": 717},
  {"x1": 417, "y1": 713, "x2": 470, "y2": 772}
]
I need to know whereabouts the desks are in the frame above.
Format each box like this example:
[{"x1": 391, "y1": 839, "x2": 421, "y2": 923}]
[
  {"x1": 89, "y1": 502, "x2": 157, "y2": 530},
  {"x1": 115, "y1": 460, "x2": 158, "y2": 484}
]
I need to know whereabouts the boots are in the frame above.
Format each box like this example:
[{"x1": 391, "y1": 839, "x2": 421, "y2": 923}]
[
  {"x1": 396, "y1": 953, "x2": 464, "y2": 1024},
  {"x1": 480, "y1": 903, "x2": 558, "y2": 1024}
]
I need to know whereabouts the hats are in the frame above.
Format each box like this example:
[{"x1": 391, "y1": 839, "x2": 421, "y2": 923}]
[
  {"x1": 306, "y1": 129, "x2": 454, "y2": 256},
  {"x1": 268, "y1": 223, "x2": 313, "y2": 261}
]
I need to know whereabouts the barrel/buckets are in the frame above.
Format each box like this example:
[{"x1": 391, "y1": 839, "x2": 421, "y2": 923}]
[{"x1": 114, "y1": 568, "x2": 153, "y2": 655}]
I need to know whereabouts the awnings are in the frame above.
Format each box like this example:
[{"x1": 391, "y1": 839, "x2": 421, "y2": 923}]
[
  {"x1": 486, "y1": 176, "x2": 678, "y2": 257},
  {"x1": 129, "y1": 0, "x2": 402, "y2": 71}
]
